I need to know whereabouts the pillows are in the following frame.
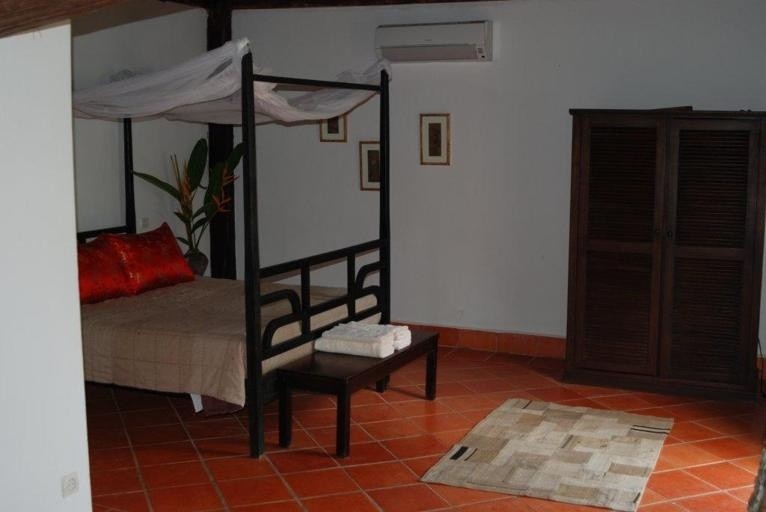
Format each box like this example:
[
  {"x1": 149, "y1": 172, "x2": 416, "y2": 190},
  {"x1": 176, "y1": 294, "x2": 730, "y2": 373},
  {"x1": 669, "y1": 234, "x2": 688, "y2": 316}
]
[{"x1": 75, "y1": 222, "x2": 195, "y2": 306}]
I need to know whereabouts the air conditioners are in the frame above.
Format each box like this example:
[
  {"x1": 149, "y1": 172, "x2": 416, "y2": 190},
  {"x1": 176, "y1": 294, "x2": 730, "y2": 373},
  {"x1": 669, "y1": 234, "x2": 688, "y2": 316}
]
[{"x1": 375, "y1": 21, "x2": 493, "y2": 63}]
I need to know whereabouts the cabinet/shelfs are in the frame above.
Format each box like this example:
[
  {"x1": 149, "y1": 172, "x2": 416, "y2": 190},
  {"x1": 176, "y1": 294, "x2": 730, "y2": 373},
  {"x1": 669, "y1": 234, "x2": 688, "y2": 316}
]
[{"x1": 562, "y1": 108, "x2": 765, "y2": 401}]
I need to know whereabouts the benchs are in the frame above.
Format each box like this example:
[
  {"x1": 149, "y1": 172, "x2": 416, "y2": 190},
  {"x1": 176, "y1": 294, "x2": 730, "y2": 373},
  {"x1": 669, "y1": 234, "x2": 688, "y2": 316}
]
[{"x1": 273, "y1": 330, "x2": 440, "y2": 459}]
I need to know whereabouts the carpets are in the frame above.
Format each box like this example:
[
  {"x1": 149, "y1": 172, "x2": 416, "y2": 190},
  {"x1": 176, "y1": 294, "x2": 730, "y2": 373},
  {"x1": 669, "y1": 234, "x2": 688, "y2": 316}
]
[{"x1": 416, "y1": 396, "x2": 676, "y2": 510}]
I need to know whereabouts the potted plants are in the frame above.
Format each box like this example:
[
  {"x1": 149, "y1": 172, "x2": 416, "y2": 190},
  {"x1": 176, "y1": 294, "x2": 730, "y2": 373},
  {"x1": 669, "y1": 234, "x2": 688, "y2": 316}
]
[{"x1": 128, "y1": 140, "x2": 245, "y2": 280}]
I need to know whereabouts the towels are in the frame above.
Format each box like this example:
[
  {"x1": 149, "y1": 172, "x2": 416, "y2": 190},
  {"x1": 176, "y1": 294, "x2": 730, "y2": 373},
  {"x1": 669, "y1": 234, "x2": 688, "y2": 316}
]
[{"x1": 313, "y1": 321, "x2": 411, "y2": 358}]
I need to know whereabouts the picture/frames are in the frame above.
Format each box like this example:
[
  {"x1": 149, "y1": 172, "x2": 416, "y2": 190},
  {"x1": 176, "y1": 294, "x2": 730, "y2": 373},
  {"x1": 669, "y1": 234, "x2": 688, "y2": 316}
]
[
  {"x1": 318, "y1": 115, "x2": 379, "y2": 190},
  {"x1": 419, "y1": 111, "x2": 451, "y2": 166}
]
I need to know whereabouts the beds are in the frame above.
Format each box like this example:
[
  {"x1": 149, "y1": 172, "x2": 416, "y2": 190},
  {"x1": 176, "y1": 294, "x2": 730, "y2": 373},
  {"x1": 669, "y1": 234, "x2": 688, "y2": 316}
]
[{"x1": 71, "y1": 54, "x2": 391, "y2": 460}]
